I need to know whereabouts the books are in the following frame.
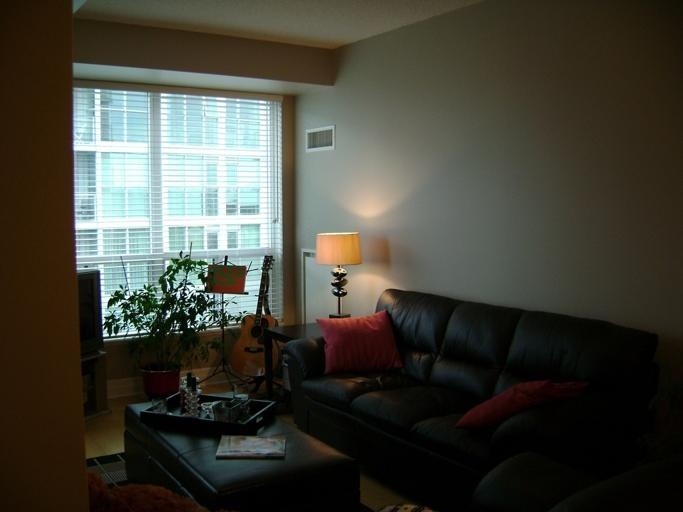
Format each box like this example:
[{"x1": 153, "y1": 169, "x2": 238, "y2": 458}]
[{"x1": 216, "y1": 435, "x2": 286, "y2": 459}]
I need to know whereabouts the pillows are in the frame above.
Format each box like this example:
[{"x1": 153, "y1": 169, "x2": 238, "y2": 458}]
[
  {"x1": 314, "y1": 310, "x2": 403, "y2": 375},
  {"x1": 457, "y1": 379, "x2": 579, "y2": 431}
]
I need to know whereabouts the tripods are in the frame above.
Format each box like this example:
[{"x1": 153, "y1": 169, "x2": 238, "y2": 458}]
[{"x1": 197, "y1": 293, "x2": 250, "y2": 392}]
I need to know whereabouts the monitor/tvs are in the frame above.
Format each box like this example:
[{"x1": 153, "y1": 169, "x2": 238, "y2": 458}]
[{"x1": 78, "y1": 269, "x2": 105, "y2": 354}]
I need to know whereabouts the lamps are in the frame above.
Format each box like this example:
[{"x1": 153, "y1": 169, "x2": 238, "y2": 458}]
[{"x1": 316, "y1": 232, "x2": 362, "y2": 319}]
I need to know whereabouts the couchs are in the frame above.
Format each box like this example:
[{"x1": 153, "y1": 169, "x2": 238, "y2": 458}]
[{"x1": 282, "y1": 289, "x2": 660, "y2": 512}]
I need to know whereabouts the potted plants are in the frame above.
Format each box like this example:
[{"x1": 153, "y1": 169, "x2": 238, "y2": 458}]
[{"x1": 103, "y1": 241, "x2": 252, "y2": 399}]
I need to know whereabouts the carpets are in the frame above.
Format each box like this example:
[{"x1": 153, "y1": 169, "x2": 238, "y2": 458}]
[{"x1": 87, "y1": 453, "x2": 374, "y2": 512}]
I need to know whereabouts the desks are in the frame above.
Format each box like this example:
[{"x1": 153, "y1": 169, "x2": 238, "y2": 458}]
[{"x1": 81, "y1": 350, "x2": 112, "y2": 423}]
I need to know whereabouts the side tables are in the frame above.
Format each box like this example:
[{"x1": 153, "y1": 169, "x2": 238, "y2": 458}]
[{"x1": 265, "y1": 324, "x2": 325, "y2": 397}]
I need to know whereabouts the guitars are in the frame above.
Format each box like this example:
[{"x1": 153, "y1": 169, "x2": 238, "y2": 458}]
[{"x1": 229, "y1": 255, "x2": 282, "y2": 376}]
